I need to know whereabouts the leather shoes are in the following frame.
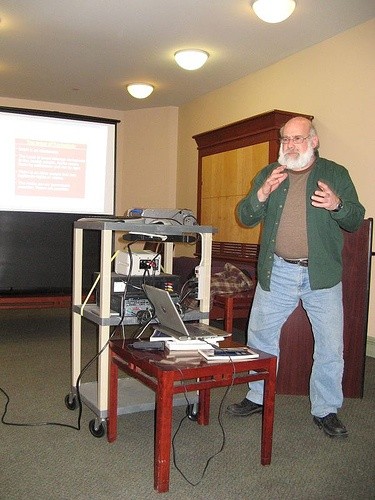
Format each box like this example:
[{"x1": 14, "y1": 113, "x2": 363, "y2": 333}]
[
  {"x1": 313, "y1": 413, "x2": 348, "y2": 438},
  {"x1": 227, "y1": 398, "x2": 264, "y2": 416}
]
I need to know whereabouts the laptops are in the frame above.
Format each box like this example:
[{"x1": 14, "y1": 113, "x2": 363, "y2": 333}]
[{"x1": 141, "y1": 284, "x2": 217, "y2": 339}]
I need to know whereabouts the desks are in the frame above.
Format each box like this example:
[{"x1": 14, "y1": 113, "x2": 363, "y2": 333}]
[
  {"x1": 107, "y1": 338, "x2": 277, "y2": 492},
  {"x1": 213, "y1": 291, "x2": 266, "y2": 341}
]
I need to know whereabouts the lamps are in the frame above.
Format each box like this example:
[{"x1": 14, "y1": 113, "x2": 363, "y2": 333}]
[
  {"x1": 173, "y1": 49, "x2": 209, "y2": 71},
  {"x1": 126, "y1": 83, "x2": 155, "y2": 99},
  {"x1": 250, "y1": 0, "x2": 296, "y2": 24}
]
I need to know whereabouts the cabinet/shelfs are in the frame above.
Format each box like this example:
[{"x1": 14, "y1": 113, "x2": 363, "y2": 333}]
[{"x1": 68, "y1": 220, "x2": 213, "y2": 416}]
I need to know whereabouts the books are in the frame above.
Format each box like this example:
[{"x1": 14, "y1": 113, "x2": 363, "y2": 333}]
[
  {"x1": 149, "y1": 346, "x2": 215, "y2": 365},
  {"x1": 202, "y1": 357, "x2": 258, "y2": 364},
  {"x1": 197, "y1": 347, "x2": 259, "y2": 361},
  {"x1": 150, "y1": 328, "x2": 225, "y2": 351}
]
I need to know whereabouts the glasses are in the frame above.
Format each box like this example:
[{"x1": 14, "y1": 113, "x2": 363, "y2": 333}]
[{"x1": 279, "y1": 135, "x2": 310, "y2": 144}]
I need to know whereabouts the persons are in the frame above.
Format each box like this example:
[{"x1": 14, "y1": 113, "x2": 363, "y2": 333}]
[{"x1": 236, "y1": 117, "x2": 367, "y2": 437}]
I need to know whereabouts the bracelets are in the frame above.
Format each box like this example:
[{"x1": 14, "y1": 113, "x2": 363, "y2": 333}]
[{"x1": 333, "y1": 197, "x2": 343, "y2": 211}]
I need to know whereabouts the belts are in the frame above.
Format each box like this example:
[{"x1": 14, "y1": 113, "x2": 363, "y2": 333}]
[{"x1": 274, "y1": 252, "x2": 308, "y2": 267}]
